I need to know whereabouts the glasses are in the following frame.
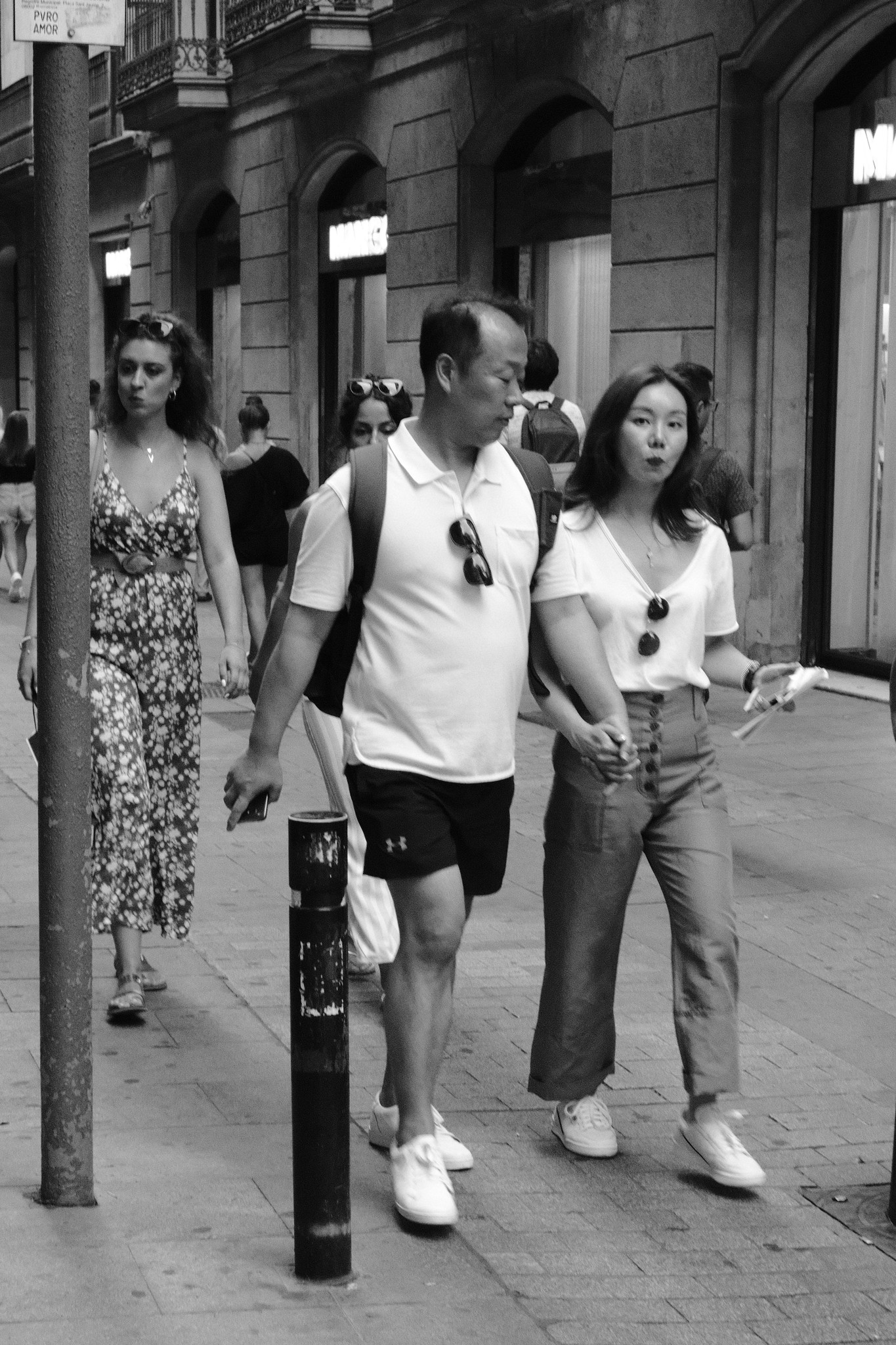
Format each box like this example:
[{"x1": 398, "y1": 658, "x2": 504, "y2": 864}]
[
  {"x1": 449, "y1": 513, "x2": 494, "y2": 588},
  {"x1": 119, "y1": 319, "x2": 175, "y2": 341},
  {"x1": 637, "y1": 592, "x2": 670, "y2": 657},
  {"x1": 706, "y1": 399, "x2": 718, "y2": 413},
  {"x1": 347, "y1": 377, "x2": 404, "y2": 398}
]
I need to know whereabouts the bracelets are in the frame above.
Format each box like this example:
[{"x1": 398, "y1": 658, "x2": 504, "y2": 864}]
[
  {"x1": 20, "y1": 636, "x2": 38, "y2": 649},
  {"x1": 743, "y1": 662, "x2": 762, "y2": 693}
]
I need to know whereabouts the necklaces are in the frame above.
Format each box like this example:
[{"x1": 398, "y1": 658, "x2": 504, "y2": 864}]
[
  {"x1": 618, "y1": 504, "x2": 660, "y2": 562},
  {"x1": 139, "y1": 444, "x2": 157, "y2": 465}
]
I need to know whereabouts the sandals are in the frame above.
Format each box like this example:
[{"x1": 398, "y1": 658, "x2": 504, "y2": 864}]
[
  {"x1": 107, "y1": 974, "x2": 148, "y2": 1016},
  {"x1": 113, "y1": 952, "x2": 167, "y2": 990}
]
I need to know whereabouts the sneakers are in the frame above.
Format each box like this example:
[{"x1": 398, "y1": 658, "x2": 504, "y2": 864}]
[
  {"x1": 390, "y1": 1132, "x2": 458, "y2": 1225},
  {"x1": 672, "y1": 1101, "x2": 767, "y2": 1187},
  {"x1": 552, "y1": 1095, "x2": 618, "y2": 1159},
  {"x1": 368, "y1": 1089, "x2": 473, "y2": 1171}
]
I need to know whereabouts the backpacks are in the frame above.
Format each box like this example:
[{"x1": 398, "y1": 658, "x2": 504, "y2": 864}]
[
  {"x1": 521, "y1": 395, "x2": 581, "y2": 464},
  {"x1": 252, "y1": 440, "x2": 560, "y2": 716}
]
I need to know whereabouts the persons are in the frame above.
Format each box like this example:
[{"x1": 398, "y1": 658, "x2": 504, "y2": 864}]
[
  {"x1": 497, "y1": 338, "x2": 587, "y2": 462},
  {"x1": 221, "y1": 297, "x2": 642, "y2": 1229},
  {"x1": 221, "y1": 395, "x2": 310, "y2": 677},
  {"x1": 0, "y1": 379, "x2": 101, "y2": 604},
  {"x1": 521, "y1": 362, "x2": 802, "y2": 1190},
  {"x1": 15, "y1": 313, "x2": 252, "y2": 1018},
  {"x1": 663, "y1": 360, "x2": 759, "y2": 554},
  {"x1": 247, "y1": 373, "x2": 400, "y2": 1008}
]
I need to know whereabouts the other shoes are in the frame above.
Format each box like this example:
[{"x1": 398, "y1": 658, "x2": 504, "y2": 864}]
[{"x1": 8, "y1": 571, "x2": 26, "y2": 601}]
